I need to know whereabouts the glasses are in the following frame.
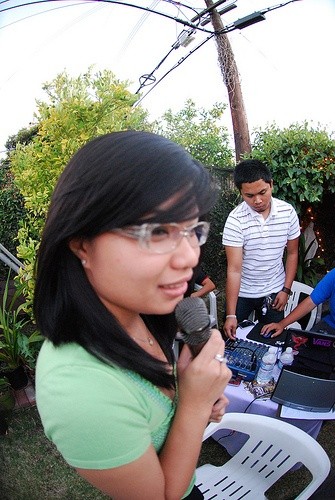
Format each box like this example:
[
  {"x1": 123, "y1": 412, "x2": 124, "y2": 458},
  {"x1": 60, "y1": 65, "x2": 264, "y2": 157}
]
[{"x1": 108, "y1": 221, "x2": 210, "y2": 255}]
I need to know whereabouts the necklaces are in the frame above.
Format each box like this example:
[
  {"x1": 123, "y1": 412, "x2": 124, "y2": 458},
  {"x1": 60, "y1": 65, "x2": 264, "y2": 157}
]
[{"x1": 130, "y1": 325, "x2": 155, "y2": 347}]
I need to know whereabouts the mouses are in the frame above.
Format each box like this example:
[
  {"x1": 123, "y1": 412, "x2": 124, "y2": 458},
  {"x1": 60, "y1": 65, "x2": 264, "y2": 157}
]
[{"x1": 263, "y1": 330, "x2": 273, "y2": 338}]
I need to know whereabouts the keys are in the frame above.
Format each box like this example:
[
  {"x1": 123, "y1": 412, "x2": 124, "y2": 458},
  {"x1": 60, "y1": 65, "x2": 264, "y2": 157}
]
[{"x1": 260, "y1": 296, "x2": 273, "y2": 312}]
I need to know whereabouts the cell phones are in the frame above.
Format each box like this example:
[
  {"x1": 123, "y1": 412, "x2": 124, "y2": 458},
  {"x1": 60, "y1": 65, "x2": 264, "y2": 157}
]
[{"x1": 239, "y1": 320, "x2": 255, "y2": 330}]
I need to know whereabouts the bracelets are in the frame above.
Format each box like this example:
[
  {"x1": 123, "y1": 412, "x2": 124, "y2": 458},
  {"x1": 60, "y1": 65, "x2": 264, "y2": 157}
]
[
  {"x1": 281, "y1": 286, "x2": 291, "y2": 295},
  {"x1": 226, "y1": 315, "x2": 237, "y2": 319}
]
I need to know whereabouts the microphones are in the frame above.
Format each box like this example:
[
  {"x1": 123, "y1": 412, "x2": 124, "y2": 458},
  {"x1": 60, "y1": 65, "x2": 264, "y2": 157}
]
[{"x1": 175, "y1": 296, "x2": 211, "y2": 356}]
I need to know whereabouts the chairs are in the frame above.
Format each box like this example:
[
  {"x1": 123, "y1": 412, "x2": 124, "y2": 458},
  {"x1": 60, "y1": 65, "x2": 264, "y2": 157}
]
[
  {"x1": 173, "y1": 284, "x2": 218, "y2": 360},
  {"x1": 253, "y1": 280, "x2": 317, "y2": 331},
  {"x1": 195, "y1": 412, "x2": 331, "y2": 500}
]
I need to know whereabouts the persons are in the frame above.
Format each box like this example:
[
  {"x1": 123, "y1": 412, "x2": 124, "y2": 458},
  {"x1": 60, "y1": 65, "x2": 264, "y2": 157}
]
[
  {"x1": 223, "y1": 160, "x2": 299, "y2": 341},
  {"x1": 260, "y1": 269, "x2": 335, "y2": 337},
  {"x1": 183, "y1": 268, "x2": 216, "y2": 299},
  {"x1": 33, "y1": 130, "x2": 229, "y2": 500}
]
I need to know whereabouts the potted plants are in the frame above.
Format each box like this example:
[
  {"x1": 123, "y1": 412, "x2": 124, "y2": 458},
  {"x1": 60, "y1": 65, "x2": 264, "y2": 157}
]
[
  {"x1": 0, "y1": 267, "x2": 33, "y2": 391},
  {"x1": 0, "y1": 376, "x2": 15, "y2": 409}
]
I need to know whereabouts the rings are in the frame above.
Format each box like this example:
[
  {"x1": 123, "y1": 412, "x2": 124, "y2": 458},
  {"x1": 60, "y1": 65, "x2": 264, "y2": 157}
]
[
  {"x1": 222, "y1": 358, "x2": 227, "y2": 363},
  {"x1": 214, "y1": 354, "x2": 222, "y2": 362}
]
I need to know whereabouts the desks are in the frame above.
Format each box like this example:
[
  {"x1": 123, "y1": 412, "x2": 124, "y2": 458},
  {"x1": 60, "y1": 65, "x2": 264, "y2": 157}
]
[{"x1": 211, "y1": 322, "x2": 323, "y2": 472}]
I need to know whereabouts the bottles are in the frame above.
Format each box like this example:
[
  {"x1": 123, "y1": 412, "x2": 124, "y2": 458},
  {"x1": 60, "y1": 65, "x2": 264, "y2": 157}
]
[
  {"x1": 257, "y1": 346, "x2": 277, "y2": 385},
  {"x1": 274, "y1": 347, "x2": 294, "y2": 382}
]
